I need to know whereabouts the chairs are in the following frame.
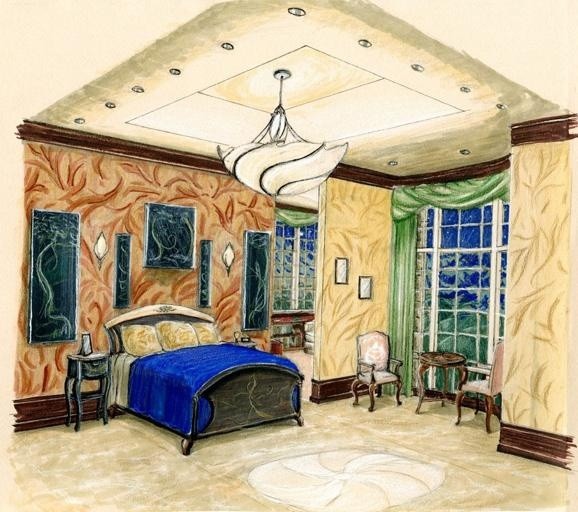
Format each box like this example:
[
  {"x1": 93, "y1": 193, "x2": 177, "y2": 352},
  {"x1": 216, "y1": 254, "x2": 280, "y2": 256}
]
[
  {"x1": 452, "y1": 336, "x2": 504, "y2": 433},
  {"x1": 350, "y1": 331, "x2": 404, "y2": 412}
]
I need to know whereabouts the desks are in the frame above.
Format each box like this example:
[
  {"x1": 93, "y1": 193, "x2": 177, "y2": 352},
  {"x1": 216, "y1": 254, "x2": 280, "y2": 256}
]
[{"x1": 415, "y1": 351, "x2": 467, "y2": 414}]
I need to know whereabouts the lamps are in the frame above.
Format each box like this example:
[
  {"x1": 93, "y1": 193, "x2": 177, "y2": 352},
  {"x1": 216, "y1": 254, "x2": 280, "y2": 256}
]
[{"x1": 216, "y1": 69, "x2": 349, "y2": 196}]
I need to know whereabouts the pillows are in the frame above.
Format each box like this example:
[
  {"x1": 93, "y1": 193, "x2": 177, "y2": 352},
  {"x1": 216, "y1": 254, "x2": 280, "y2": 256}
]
[{"x1": 120, "y1": 320, "x2": 220, "y2": 355}]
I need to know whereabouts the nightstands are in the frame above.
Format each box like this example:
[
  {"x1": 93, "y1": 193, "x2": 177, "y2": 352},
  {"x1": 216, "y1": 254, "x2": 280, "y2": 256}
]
[{"x1": 64, "y1": 352, "x2": 112, "y2": 431}]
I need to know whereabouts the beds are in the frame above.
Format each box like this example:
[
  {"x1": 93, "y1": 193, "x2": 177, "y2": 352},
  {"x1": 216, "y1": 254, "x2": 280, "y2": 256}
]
[{"x1": 103, "y1": 303, "x2": 305, "y2": 455}]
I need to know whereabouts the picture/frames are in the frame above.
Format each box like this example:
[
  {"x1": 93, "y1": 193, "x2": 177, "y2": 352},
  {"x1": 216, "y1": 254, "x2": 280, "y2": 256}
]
[
  {"x1": 77, "y1": 333, "x2": 93, "y2": 356},
  {"x1": 333, "y1": 257, "x2": 373, "y2": 299},
  {"x1": 26, "y1": 202, "x2": 272, "y2": 346}
]
